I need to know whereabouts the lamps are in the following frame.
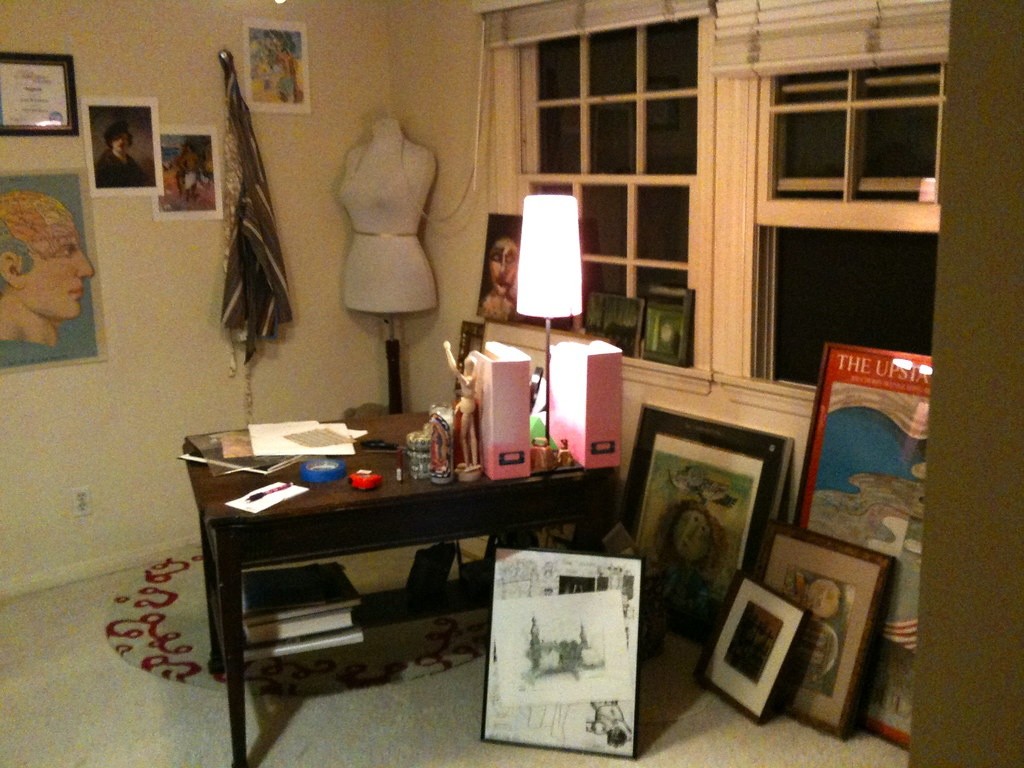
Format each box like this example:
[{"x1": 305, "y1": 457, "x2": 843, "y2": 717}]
[{"x1": 515, "y1": 193, "x2": 584, "y2": 474}]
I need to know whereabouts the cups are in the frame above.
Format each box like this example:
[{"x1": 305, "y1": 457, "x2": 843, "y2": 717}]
[{"x1": 408, "y1": 431, "x2": 429, "y2": 479}]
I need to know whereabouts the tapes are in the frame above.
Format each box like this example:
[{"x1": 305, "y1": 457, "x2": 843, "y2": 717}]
[{"x1": 300, "y1": 459, "x2": 346, "y2": 482}]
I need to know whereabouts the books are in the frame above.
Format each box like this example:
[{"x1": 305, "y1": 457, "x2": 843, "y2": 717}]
[{"x1": 237, "y1": 564, "x2": 364, "y2": 661}]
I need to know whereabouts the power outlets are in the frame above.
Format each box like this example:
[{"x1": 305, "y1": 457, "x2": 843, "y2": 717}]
[{"x1": 72, "y1": 486, "x2": 92, "y2": 517}]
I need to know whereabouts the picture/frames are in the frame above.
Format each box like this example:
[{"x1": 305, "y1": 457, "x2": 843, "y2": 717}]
[
  {"x1": 0, "y1": 52, "x2": 80, "y2": 136},
  {"x1": 456, "y1": 287, "x2": 932, "y2": 761}
]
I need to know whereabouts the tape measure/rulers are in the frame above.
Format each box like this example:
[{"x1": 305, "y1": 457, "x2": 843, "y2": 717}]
[{"x1": 348, "y1": 469, "x2": 384, "y2": 490}]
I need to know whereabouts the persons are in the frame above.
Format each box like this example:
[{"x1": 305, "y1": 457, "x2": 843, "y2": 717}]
[
  {"x1": 338, "y1": 116, "x2": 439, "y2": 313},
  {"x1": 95, "y1": 121, "x2": 146, "y2": 187},
  {"x1": 442, "y1": 341, "x2": 478, "y2": 469}
]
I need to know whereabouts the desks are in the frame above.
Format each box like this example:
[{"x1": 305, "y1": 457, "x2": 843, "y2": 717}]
[{"x1": 181, "y1": 411, "x2": 629, "y2": 768}]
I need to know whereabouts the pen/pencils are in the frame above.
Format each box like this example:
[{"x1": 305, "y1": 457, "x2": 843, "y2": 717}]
[
  {"x1": 396, "y1": 449, "x2": 402, "y2": 481},
  {"x1": 246, "y1": 482, "x2": 293, "y2": 501}
]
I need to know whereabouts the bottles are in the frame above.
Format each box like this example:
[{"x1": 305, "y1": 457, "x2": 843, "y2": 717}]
[{"x1": 428, "y1": 401, "x2": 455, "y2": 485}]
